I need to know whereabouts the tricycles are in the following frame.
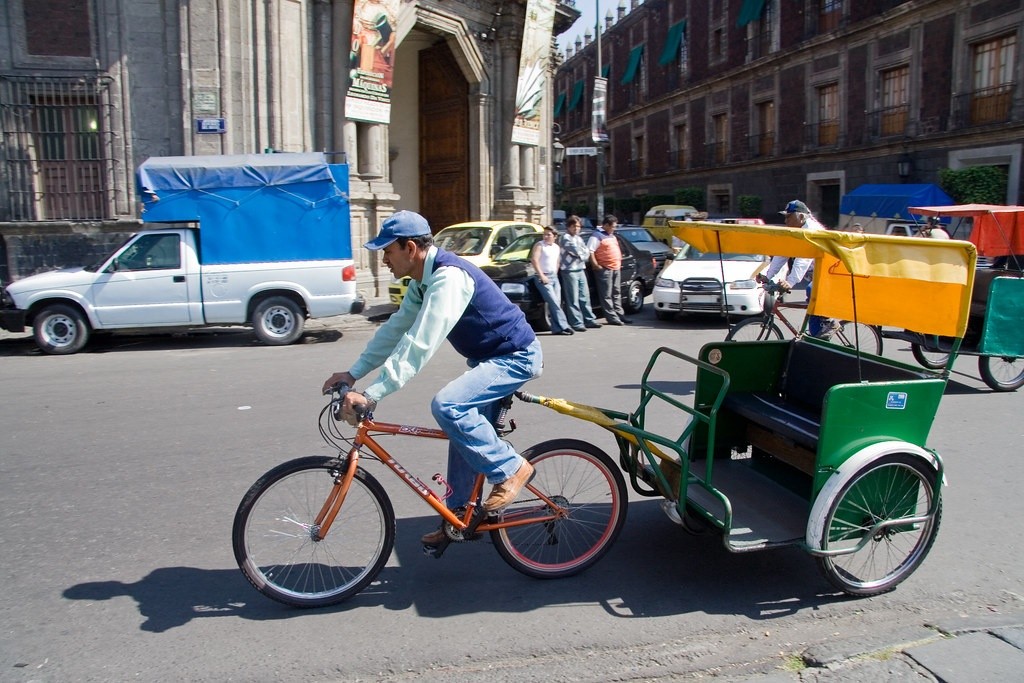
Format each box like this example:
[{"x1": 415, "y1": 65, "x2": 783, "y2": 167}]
[
  {"x1": 903, "y1": 203, "x2": 1024, "y2": 278},
  {"x1": 231, "y1": 218, "x2": 980, "y2": 611},
  {"x1": 722, "y1": 203, "x2": 1024, "y2": 393}
]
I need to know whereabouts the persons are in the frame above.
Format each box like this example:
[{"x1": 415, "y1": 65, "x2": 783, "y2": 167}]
[
  {"x1": 756, "y1": 201, "x2": 841, "y2": 341},
  {"x1": 558, "y1": 215, "x2": 602, "y2": 332},
  {"x1": 322, "y1": 211, "x2": 544, "y2": 543},
  {"x1": 587, "y1": 214, "x2": 633, "y2": 326},
  {"x1": 913, "y1": 216, "x2": 951, "y2": 239},
  {"x1": 530, "y1": 225, "x2": 575, "y2": 335}
]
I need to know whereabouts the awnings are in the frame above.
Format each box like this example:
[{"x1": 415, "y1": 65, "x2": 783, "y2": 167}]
[
  {"x1": 620, "y1": 43, "x2": 642, "y2": 85},
  {"x1": 554, "y1": 91, "x2": 566, "y2": 119},
  {"x1": 569, "y1": 78, "x2": 584, "y2": 114},
  {"x1": 591, "y1": 64, "x2": 610, "y2": 101},
  {"x1": 736, "y1": 0, "x2": 765, "y2": 28},
  {"x1": 659, "y1": 18, "x2": 686, "y2": 64}
]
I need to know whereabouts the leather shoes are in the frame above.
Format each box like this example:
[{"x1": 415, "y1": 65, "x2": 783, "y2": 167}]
[
  {"x1": 484, "y1": 459, "x2": 536, "y2": 510},
  {"x1": 421, "y1": 527, "x2": 482, "y2": 545}
]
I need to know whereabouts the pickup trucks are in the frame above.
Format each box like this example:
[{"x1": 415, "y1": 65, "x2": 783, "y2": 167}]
[{"x1": 0, "y1": 227, "x2": 365, "y2": 355}]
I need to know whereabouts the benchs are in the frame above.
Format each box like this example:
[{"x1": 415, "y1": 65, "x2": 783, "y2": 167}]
[
  {"x1": 967, "y1": 267, "x2": 1024, "y2": 319},
  {"x1": 725, "y1": 342, "x2": 937, "y2": 452}
]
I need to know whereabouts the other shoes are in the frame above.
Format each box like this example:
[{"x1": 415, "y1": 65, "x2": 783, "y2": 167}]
[
  {"x1": 586, "y1": 323, "x2": 602, "y2": 328},
  {"x1": 820, "y1": 318, "x2": 842, "y2": 340},
  {"x1": 619, "y1": 317, "x2": 632, "y2": 324},
  {"x1": 576, "y1": 327, "x2": 586, "y2": 332},
  {"x1": 616, "y1": 321, "x2": 624, "y2": 325},
  {"x1": 554, "y1": 327, "x2": 573, "y2": 337}
]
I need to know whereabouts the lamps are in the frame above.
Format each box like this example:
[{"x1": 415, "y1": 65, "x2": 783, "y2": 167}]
[{"x1": 897, "y1": 154, "x2": 915, "y2": 183}]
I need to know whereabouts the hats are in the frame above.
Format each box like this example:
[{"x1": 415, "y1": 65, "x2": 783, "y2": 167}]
[
  {"x1": 778, "y1": 200, "x2": 810, "y2": 215},
  {"x1": 363, "y1": 210, "x2": 431, "y2": 250}
]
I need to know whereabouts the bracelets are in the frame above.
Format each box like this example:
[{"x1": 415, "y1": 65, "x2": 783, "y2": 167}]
[{"x1": 363, "y1": 392, "x2": 376, "y2": 406}]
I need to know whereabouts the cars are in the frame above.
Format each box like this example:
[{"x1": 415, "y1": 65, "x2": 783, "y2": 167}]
[
  {"x1": 387, "y1": 223, "x2": 545, "y2": 308},
  {"x1": 592, "y1": 224, "x2": 675, "y2": 277},
  {"x1": 651, "y1": 244, "x2": 787, "y2": 324},
  {"x1": 557, "y1": 217, "x2": 596, "y2": 230},
  {"x1": 474, "y1": 225, "x2": 656, "y2": 331}
]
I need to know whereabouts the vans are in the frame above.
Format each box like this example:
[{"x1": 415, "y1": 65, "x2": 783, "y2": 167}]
[{"x1": 641, "y1": 204, "x2": 698, "y2": 250}]
